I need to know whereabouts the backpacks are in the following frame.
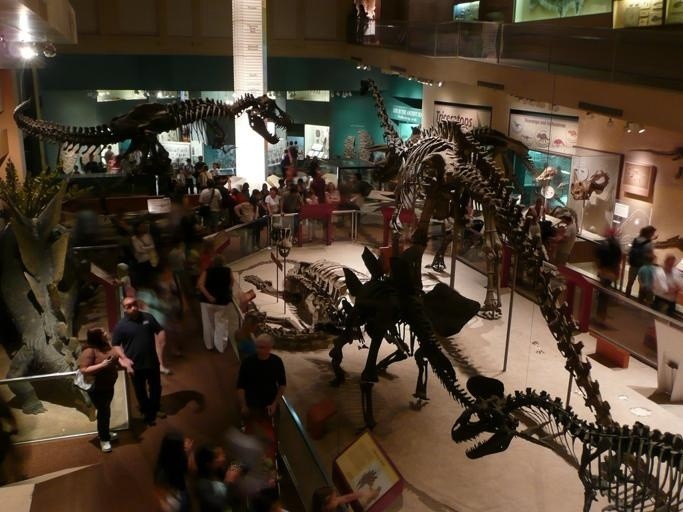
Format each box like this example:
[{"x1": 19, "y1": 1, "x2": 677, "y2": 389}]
[{"x1": 627, "y1": 238, "x2": 652, "y2": 268}]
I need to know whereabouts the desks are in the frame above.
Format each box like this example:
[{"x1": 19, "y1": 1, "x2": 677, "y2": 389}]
[{"x1": 319, "y1": 158, "x2": 382, "y2": 193}]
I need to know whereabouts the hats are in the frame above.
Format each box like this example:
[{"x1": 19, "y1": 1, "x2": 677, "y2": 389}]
[
  {"x1": 655, "y1": 247, "x2": 682, "y2": 269},
  {"x1": 558, "y1": 212, "x2": 573, "y2": 220}
]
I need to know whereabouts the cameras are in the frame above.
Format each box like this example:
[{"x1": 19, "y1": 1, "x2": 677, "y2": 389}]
[{"x1": 107, "y1": 356, "x2": 118, "y2": 369}]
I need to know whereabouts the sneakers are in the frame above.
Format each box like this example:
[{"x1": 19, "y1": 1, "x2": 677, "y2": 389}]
[{"x1": 98, "y1": 431, "x2": 120, "y2": 453}]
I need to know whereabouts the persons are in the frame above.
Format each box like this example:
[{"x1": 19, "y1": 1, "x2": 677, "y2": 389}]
[
  {"x1": 110, "y1": 298, "x2": 168, "y2": 423},
  {"x1": 152, "y1": 335, "x2": 378, "y2": 512},
  {"x1": 80, "y1": 325, "x2": 126, "y2": 452},
  {"x1": 626, "y1": 225, "x2": 657, "y2": 301},
  {"x1": 176, "y1": 150, "x2": 373, "y2": 251},
  {"x1": 526, "y1": 197, "x2": 577, "y2": 268},
  {"x1": 593, "y1": 225, "x2": 622, "y2": 328},
  {"x1": 636, "y1": 253, "x2": 658, "y2": 306},
  {"x1": 0, "y1": 394, "x2": 28, "y2": 486},
  {"x1": 115, "y1": 217, "x2": 234, "y2": 375},
  {"x1": 651, "y1": 255, "x2": 683, "y2": 319},
  {"x1": 287, "y1": 141, "x2": 298, "y2": 168},
  {"x1": 86, "y1": 146, "x2": 113, "y2": 171}
]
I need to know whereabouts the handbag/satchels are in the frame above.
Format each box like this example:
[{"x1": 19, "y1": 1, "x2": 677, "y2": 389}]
[
  {"x1": 195, "y1": 205, "x2": 211, "y2": 217},
  {"x1": 74, "y1": 369, "x2": 95, "y2": 391},
  {"x1": 148, "y1": 246, "x2": 161, "y2": 268}
]
